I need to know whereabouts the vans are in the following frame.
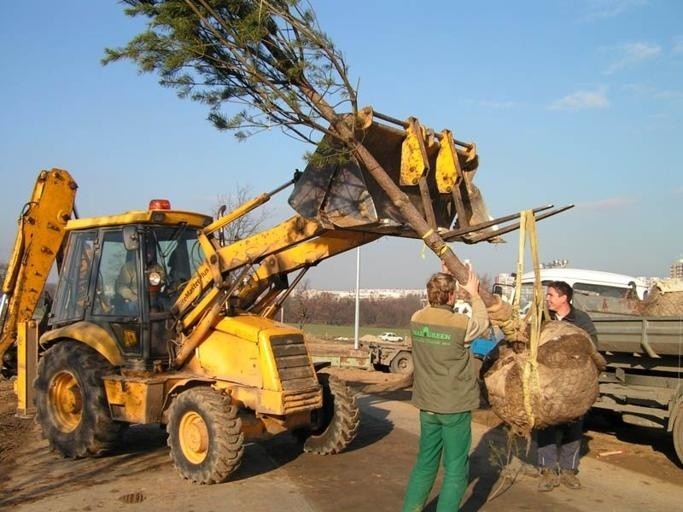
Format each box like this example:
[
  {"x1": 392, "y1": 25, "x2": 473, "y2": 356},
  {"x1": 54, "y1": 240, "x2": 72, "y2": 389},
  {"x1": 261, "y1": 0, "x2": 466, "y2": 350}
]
[{"x1": 492, "y1": 259, "x2": 648, "y2": 326}]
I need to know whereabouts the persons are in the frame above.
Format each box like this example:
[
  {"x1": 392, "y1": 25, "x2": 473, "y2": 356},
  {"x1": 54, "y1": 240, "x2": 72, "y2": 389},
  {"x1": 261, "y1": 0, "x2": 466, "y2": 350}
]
[
  {"x1": 116, "y1": 239, "x2": 165, "y2": 315},
  {"x1": 536, "y1": 281, "x2": 598, "y2": 491},
  {"x1": 402, "y1": 259, "x2": 489, "y2": 512}
]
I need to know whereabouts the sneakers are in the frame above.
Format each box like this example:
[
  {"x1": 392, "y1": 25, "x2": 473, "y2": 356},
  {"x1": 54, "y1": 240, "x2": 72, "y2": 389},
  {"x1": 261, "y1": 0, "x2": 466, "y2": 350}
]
[
  {"x1": 538, "y1": 471, "x2": 562, "y2": 491},
  {"x1": 560, "y1": 469, "x2": 582, "y2": 489}
]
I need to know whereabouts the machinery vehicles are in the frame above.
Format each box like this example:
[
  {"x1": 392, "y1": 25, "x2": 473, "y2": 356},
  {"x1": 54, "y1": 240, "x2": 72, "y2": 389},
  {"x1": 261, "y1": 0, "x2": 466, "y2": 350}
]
[{"x1": 573, "y1": 292, "x2": 683, "y2": 466}]
